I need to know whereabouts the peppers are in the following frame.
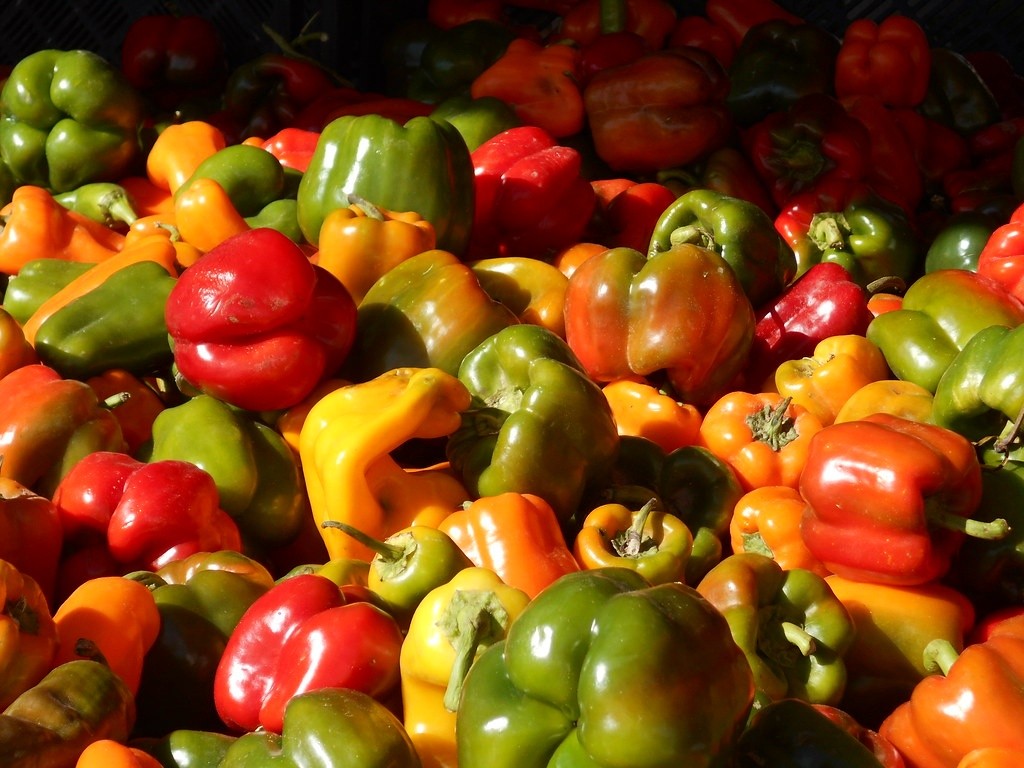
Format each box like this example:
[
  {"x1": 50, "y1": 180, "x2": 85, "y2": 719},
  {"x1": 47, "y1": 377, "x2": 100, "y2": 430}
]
[{"x1": 0, "y1": 0, "x2": 1024, "y2": 768}]
